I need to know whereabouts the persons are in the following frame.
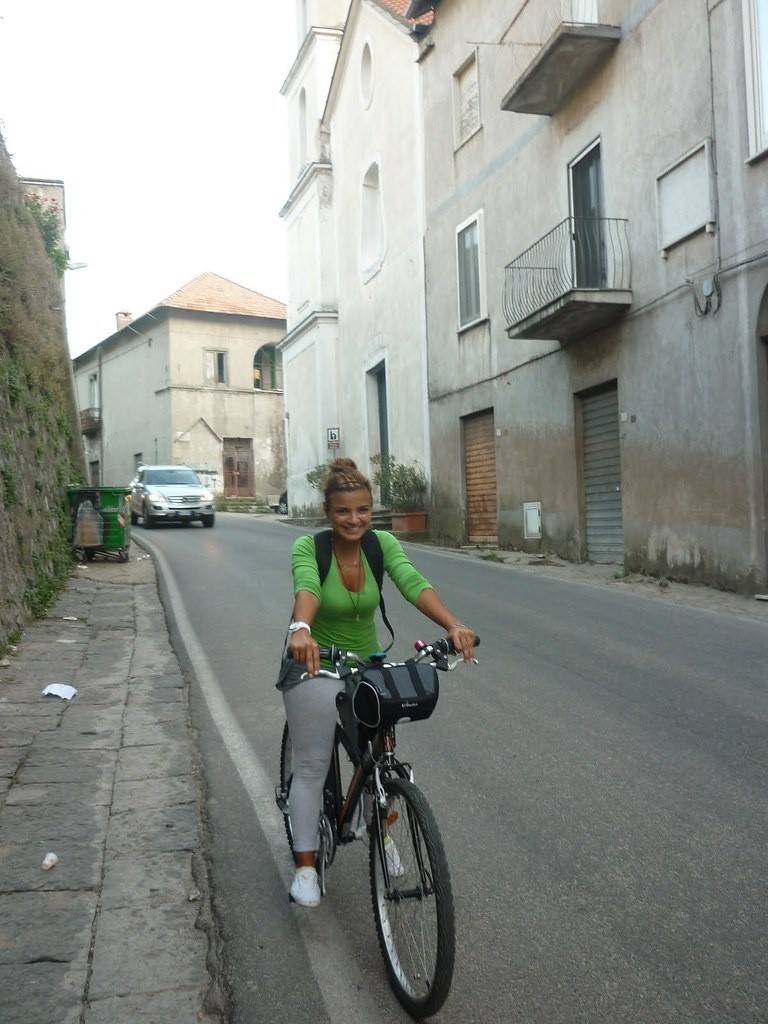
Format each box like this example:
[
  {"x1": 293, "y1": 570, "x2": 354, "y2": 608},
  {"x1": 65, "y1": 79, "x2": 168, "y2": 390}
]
[{"x1": 272, "y1": 457, "x2": 478, "y2": 912}]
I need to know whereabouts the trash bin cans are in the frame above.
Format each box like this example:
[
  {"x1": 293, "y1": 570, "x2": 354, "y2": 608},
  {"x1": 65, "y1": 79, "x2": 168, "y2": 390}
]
[{"x1": 65, "y1": 482, "x2": 134, "y2": 551}]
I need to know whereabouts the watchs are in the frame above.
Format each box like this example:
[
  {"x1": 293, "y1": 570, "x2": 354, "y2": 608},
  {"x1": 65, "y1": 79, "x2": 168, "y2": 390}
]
[{"x1": 286, "y1": 620, "x2": 312, "y2": 641}]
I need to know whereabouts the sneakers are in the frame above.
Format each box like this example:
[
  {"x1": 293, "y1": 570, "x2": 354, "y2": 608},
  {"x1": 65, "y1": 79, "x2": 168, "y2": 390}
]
[
  {"x1": 362, "y1": 826, "x2": 404, "y2": 876},
  {"x1": 290, "y1": 870, "x2": 321, "y2": 906}
]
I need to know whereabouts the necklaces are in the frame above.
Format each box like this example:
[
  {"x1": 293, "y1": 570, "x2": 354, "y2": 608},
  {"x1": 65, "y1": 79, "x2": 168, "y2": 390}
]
[
  {"x1": 331, "y1": 538, "x2": 362, "y2": 622},
  {"x1": 333, "y1": 534, "x2": 361, "y2": 574}
]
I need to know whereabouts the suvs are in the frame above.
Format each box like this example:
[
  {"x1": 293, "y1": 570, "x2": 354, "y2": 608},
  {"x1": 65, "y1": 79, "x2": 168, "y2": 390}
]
[{"x1": 130, "y1": 464, "x2": 215, "y2": 527}]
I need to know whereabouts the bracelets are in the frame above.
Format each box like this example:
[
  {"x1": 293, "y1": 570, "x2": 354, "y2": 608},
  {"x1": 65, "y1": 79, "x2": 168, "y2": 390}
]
[{"x1": 445, "y1": 624, "x2": 467, "y2": 631}]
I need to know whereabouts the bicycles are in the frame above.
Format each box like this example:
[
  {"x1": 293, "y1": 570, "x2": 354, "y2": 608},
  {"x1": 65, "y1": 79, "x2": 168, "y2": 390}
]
[{"x1": 277, "y1": 632, "x2": 481, "y2": 1024}]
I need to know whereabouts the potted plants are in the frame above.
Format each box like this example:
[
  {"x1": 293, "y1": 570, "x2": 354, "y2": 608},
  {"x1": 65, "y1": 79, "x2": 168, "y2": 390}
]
[{"x1": 370, "y1": 453, "x2": 429, "y2": 532}]
